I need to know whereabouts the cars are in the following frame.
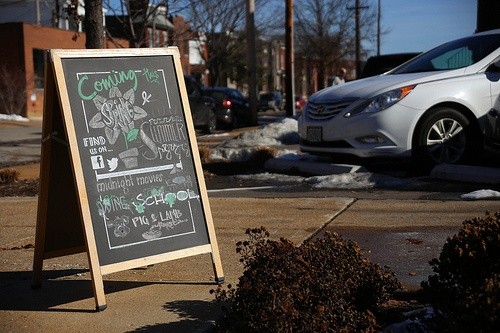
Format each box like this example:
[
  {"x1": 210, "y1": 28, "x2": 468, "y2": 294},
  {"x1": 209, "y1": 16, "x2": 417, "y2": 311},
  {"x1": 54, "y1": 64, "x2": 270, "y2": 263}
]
[
  {"x1": 259, "y1": 93, "x2": 281, "y2": 111},
  {"x1": 294, "y1": 95, "x2": 305, "y2": 110},
  {"x1": 297, "y1": 28, "x2": 500, "y2": 176},
  {"x1": 183, "y1": 74, "x2": 217, "y2": 132},
  {"x1": 202, "y1": 86, "x2": 250, "y2": 127}
]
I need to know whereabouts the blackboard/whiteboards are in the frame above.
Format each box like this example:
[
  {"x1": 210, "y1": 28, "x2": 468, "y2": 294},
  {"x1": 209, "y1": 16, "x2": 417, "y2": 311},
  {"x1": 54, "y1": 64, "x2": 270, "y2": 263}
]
[{"x1": 36, "y1": 48, "x2": 211, "y2": 275}]
